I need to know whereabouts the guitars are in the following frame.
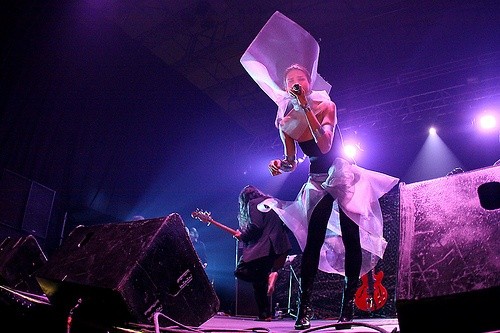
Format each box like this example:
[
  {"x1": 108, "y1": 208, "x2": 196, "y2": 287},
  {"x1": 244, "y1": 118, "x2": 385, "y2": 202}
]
[
  {"x1": 190, "y1": 208, "x2": 237, "y2": 235},
  {"x1": 355, "y1": 252, "x2": 387, "y2": 312}
]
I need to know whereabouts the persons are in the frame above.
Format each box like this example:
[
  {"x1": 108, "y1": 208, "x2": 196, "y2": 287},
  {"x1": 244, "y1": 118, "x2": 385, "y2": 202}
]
[
  {"x1": 268, "y1": 64, "x2": 363, "y2": 330},
  {"x1": 233, "y1": 184, "x2": 302, "y2": 321}
]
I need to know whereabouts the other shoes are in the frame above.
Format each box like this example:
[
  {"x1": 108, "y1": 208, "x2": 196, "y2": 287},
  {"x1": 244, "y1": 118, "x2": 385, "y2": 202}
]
[{"x1": 253, "y1": 317, "x2": 271, "y2": 322}]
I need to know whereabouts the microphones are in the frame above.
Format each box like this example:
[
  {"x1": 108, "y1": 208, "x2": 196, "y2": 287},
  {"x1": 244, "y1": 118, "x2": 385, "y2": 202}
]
[{"x1": 292, "y1": 84, "x2": 301, "y2": 94}]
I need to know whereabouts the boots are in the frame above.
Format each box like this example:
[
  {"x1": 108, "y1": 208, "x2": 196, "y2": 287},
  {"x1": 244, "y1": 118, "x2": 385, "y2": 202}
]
[
  {"x1": 294, "y1": 277, "x2": 314, "y2": 329},
  {"x1": 335, "y1": 276, "x2": 362, "y2": 329}
]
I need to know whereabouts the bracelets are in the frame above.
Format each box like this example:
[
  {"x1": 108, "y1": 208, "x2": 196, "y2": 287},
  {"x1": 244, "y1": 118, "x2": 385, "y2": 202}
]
[
  {"x1": 304, "y1": 106, "x2": 311, "y2": 112},
  {"x1": 281, "y1": 161, "x2": 293, "y2": 172}
]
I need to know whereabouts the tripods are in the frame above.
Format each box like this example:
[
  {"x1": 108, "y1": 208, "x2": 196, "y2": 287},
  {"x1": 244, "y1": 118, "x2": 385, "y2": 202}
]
[{"x1": 271, "y1": 263, "x2": 305, "y2": 320}]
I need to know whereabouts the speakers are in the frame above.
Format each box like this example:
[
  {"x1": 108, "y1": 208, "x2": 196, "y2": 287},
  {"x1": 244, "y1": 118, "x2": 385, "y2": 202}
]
[
  {"x1": 236, "y1": 240, "x2": 271, "y2": 320},
  {"x1": 0, "y1": 235, "x2": 56, "y2": 333},
  {"x1": 48, "y1": 211, "x2": 221, "y2": 331},
  {"x1": 394, "y1": 165, "x2": 500, "y2": 333}
]
